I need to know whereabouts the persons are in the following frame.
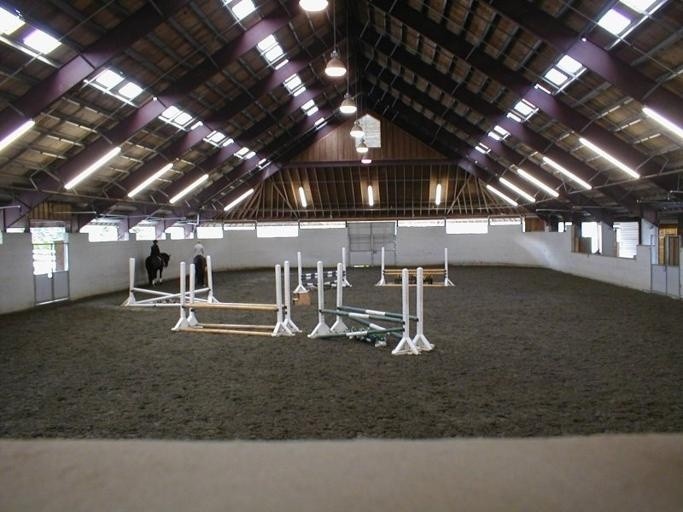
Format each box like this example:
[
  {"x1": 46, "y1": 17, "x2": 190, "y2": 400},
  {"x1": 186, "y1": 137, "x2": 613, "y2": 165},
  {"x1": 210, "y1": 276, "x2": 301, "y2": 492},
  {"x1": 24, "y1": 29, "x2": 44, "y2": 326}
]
[
  {"x1": 192, "y1": 241, "x2": 207, "y2": 266},
  {"x1": 150, "y1": 240, "x2": 164, "y2": 266}
]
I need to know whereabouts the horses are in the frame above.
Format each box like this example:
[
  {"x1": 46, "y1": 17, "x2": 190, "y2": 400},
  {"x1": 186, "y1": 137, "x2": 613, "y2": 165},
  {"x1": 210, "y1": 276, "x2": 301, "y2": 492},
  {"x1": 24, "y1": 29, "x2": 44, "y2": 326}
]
[
  {"x1": 145, "y1": 253, "x2": 171, "y2": 288},
  {"x1": 193, "y1": 255, "x2": 207, "y2": 288}
]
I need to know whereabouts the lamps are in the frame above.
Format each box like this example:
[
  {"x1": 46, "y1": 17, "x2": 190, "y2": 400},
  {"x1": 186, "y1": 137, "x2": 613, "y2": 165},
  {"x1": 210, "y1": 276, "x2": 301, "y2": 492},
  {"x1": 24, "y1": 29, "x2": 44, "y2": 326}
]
[
  {"x1": 297, "y1": 184, "x2": 308, "y2": 208},
  {"x1": 298, "y1": 0, "x2": 373, "y2": 168},
  {"x1": 365, "y1": 183, "x2": 374, "y2": 207},
  {"x1": 480, "y1": 106, "x2": 682, "y2": 210},
  {"x1": 433, "y1": 181, "x2": 442, "y2": 206},
  {"x1": 0, "y1": 114, "x2": 257, "y2": 215}
]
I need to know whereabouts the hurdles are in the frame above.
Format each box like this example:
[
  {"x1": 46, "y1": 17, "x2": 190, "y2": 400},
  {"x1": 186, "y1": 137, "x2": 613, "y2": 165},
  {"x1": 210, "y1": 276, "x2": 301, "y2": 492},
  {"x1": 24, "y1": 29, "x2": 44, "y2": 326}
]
[
  {"x1": 116, "y1": 254, "x2": 301, "y2": 338},
  {"x1": 291, "y1": 243, "x2": 459, "y2": 357}
]
